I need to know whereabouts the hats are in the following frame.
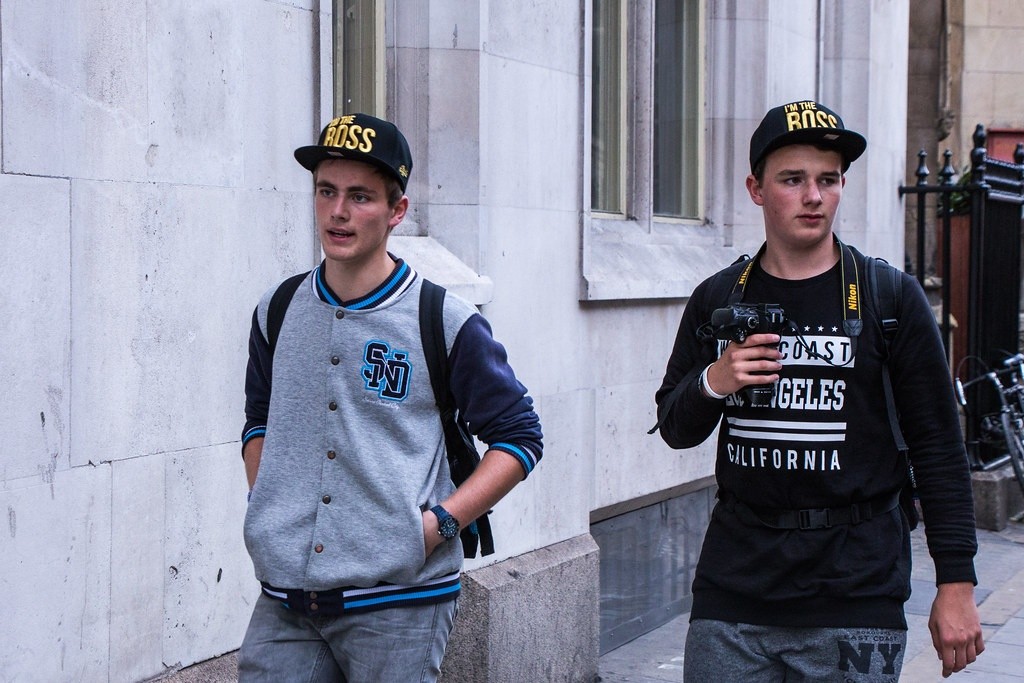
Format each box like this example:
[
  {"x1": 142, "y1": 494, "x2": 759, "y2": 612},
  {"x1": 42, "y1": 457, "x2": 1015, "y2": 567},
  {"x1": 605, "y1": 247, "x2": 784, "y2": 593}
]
[
  {"x1": 292, "y1": 114, "x2": 413, "y2": 195},
  {"x1": 749, "y1": 100, "x2": 868, "y2": 173}
]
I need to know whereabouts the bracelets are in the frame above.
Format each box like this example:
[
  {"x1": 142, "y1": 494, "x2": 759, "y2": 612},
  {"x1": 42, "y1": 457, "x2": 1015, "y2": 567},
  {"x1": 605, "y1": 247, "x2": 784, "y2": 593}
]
[{"x1": 703, "y1": 363, "x2": 729, "y2": 398}]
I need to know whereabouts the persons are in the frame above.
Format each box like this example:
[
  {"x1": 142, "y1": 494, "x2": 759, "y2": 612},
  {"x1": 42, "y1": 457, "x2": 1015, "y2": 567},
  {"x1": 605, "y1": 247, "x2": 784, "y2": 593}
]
[
  {"x1": 235, "y1": 112, "x2": 544, "y2": 683},
  {"x1": 653, "y1": 99, "x2": 985, "y2": 683}
]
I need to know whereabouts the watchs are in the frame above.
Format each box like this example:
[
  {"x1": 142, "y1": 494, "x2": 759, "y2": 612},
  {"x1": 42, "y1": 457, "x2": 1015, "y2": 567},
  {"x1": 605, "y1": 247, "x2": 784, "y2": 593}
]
[{"x1": 430, "y1": 505, "x2": 461, "y2": 541}]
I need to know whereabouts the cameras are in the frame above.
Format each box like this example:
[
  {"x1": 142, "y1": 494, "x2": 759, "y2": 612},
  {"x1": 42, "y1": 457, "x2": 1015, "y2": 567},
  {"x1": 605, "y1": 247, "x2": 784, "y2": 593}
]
[{"x1": 711, "y1": 303, "x2": 785, "y2": 405}]
